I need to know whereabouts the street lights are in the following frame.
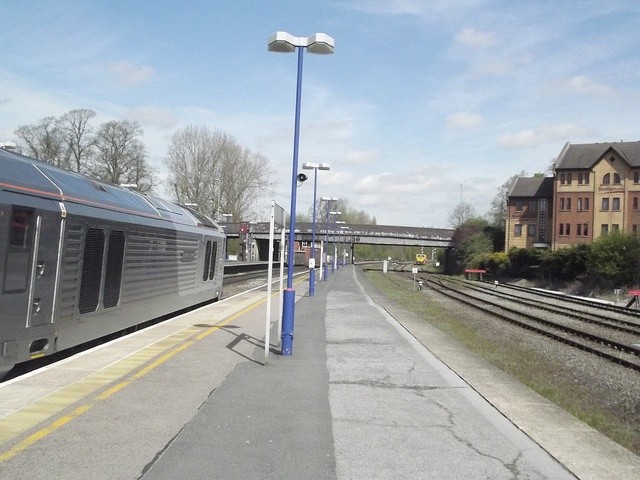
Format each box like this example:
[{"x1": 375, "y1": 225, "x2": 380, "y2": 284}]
[
  {"x1": 335, "y1": 220, "x2": 345, "y2": 270},
  {"x1": 321, "y1": 197, "x2": 337, "y2": 282},
  {"x1": 301, "y1": 161, "x2": 330, "y2": 297},
  {"x1": 265, "y1": 29, "x2": 336, "y2": 358},
  {"x1": 341, "y1": 226, "x2": 349, "y2": 267},
  {"x1": 330, "y1": 211, "x2": 341, "y2": 275}
]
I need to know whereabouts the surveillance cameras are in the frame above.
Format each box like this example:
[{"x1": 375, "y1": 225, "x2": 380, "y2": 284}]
[{"x1": 297, "y1": 173, "x2": 308, "y2": 182}]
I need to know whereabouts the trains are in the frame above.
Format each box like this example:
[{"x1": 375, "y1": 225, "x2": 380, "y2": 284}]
[
  {"x1": 416, "y1": 253, "x2": 428, "y2": 265},
  {"x1": 1, "y1": 138, "x2": 226, "y2": 384}
]
